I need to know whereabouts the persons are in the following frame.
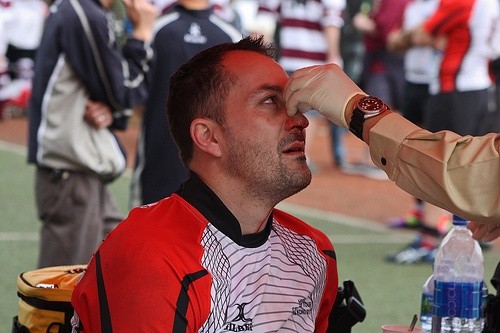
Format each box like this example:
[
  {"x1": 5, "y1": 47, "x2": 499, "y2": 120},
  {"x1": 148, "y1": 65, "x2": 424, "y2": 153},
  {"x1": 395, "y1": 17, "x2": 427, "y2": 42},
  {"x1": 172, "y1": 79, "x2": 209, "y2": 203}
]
[
  {"x1": 68, "y1": 33, "x2": 338, "y2": 333},
  {"x1": 282, "y1": 63, "x2": 500, "y2": 243},
  {"x1": 0, "y1": 1, "x2": 499, "y2": 268}
]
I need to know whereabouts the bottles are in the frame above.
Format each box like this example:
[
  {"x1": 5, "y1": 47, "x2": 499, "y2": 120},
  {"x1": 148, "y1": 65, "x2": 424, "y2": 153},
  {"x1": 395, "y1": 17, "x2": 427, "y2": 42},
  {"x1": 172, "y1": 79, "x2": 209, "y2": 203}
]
[
  {"x1": 419, "y1": 272, "x2": 434, "y2": 333},
  {"x1": 431, "y1": 214, "x2": 484, "y2": 333},
  {"x1": 482, "y1": 280, "x2": 488, "y2": 327}
]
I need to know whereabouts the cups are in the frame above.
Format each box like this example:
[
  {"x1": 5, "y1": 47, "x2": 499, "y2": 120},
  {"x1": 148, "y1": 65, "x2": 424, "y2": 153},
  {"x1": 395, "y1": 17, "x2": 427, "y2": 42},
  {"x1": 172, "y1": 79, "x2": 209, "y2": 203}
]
[{"x1": 382, "y1": 324, "x2": 422, "y2": 333}]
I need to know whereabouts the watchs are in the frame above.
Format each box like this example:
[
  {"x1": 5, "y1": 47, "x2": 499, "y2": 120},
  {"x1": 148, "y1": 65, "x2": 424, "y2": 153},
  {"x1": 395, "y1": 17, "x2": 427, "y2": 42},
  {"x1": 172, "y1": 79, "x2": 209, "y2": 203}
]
[{"x1": 349, "y1": 95, "x2": 391, "y2": 140}]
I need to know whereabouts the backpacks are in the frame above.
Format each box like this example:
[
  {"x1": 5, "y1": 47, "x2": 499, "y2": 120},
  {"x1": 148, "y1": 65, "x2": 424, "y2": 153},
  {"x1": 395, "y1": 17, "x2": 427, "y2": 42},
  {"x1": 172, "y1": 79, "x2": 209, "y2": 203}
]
[{"x1": 11, "y1": 265, "x2": 88, "y2": 333}]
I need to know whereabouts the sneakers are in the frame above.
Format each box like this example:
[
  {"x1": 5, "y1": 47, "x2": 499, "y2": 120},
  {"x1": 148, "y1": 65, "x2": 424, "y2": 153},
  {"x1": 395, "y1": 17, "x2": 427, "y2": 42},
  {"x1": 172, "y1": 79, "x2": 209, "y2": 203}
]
[
  {"x1": 385, "y1": 215, "x2": 422, "y2": 229},
  {"x1": 340, "y1": 158, "x2": 389, "y2": 181},
  {"x1": 384, "y1": 241, "x2": 440, "y2": 265}
]
